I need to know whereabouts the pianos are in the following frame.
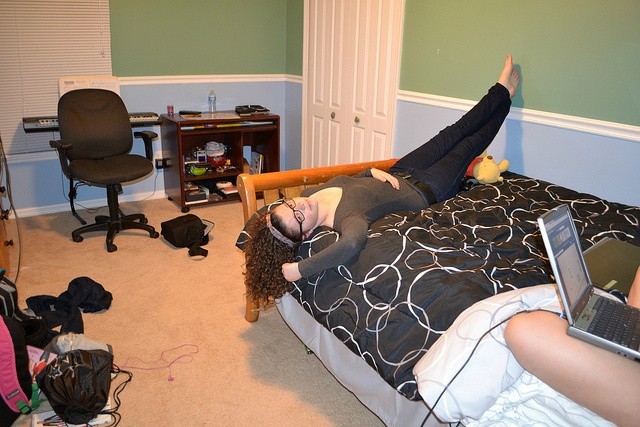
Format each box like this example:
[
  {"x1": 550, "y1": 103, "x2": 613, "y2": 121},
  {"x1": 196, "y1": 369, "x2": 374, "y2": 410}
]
[{"x1": 21, "y1": 113, "x2": 163, "y2": 133}]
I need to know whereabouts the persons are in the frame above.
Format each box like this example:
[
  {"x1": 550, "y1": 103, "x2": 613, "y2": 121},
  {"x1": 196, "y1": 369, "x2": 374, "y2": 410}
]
[
  {"x1": 503, "y1": 264, "x2": 640, "y2": 427},
  {"x1": 241, "y1": 55, "x2": 520, "y2": 312}
]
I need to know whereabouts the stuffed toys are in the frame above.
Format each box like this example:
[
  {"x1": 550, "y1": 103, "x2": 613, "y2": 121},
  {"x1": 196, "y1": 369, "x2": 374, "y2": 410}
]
[{"x1": 465, "y1": 150, "x2": 510, "y2": 184}]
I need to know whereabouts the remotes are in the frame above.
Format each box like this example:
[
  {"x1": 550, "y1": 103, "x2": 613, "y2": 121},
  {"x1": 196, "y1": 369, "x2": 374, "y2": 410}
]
[{"x1": 179, "y1": 110, "x2": 202, "y2": 114}]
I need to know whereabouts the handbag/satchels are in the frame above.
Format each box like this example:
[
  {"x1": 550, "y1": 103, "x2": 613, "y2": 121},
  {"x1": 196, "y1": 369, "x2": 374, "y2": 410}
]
[
  {"x1": 36, "y1": 348, "x2": 113, "y2": 424},
  {"x1": 161, "y1": 214, "x2": 214, "y2": 248}
]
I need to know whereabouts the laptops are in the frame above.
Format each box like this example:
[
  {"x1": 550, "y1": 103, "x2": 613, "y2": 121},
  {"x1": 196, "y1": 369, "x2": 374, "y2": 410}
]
[{"x1": 537, "y1": 204, "x2": 639, "y2": 365}]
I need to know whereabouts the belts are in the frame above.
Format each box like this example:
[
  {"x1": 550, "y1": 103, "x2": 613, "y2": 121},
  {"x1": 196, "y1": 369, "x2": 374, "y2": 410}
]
[{"x1": 396, "y1": 171, "x2": 437, "y2": 204}]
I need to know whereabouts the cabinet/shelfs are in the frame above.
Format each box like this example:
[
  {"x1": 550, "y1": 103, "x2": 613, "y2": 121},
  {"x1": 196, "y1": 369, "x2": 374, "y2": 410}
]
[{"x1": 160, "y1": 110, "x2": 280, "y2": 213}]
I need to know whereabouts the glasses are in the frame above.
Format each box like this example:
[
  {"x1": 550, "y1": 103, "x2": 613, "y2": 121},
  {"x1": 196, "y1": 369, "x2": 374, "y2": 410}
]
[{"x1": 282, "y1": 198, "x2": 304, "y2": 241}]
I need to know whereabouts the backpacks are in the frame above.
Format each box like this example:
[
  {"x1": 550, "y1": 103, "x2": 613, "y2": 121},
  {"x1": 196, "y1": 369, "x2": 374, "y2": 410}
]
[{"x1": 0, "y1": 312, "x2": 40, "y2": 427}]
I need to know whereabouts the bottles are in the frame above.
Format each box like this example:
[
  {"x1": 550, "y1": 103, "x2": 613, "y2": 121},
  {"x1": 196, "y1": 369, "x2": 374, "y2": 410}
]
[{"x1": 208, "y1": 88, "x2": 218, "y2": 113}]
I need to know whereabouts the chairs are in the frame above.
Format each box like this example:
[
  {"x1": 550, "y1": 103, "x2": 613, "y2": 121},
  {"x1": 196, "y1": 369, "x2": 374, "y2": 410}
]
[{"x1": 49, "y1": 88, "x2": 159, "y2": 252}]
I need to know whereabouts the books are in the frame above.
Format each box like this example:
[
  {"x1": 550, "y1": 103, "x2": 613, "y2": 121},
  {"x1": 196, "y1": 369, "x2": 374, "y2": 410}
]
[
  {"x1": 251, "y1": 151, "x2": 265, "y2": 174},
  {"x1": 248, "y1": 97, "x2": 269, "y2": 114},
  {"x1": 219, "y1": 186, "x2": 239, "y2": 195},
  {"x1": 229, "y1": 103, "x2": 252, "y2": 116}
]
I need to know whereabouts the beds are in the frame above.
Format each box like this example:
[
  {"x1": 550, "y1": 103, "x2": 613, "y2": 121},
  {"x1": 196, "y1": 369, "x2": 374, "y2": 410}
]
[{"x1": 237, "y1": 159, "x2": 640, "y2": 427}]
